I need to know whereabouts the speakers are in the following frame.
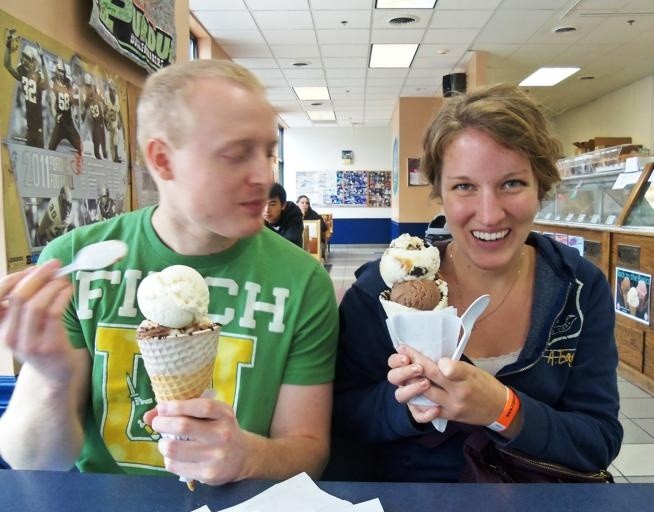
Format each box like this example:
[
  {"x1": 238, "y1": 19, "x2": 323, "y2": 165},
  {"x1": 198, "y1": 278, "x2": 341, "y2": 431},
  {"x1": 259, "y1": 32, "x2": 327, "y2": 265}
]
[{"x1": 443, "y1": 73, "x2": 466, "y2": 97}]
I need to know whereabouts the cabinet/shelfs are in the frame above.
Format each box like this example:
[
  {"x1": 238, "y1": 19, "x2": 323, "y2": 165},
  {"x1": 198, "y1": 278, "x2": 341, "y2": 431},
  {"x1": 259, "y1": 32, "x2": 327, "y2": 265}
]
[{"x1": 533, "y1": 146, "x2": 654, "y2": 395}]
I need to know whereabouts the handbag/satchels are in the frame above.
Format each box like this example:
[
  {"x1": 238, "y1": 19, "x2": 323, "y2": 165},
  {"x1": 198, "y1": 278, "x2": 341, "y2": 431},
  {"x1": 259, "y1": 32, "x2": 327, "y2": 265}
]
[{"x1": 342, "y1": 420, "x2": 615, "y2": 483}]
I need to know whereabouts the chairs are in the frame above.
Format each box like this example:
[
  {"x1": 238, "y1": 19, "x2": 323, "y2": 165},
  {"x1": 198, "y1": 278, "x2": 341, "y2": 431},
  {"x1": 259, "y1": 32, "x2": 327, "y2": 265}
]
[{"x1": 300, "y1": 214, "x2": 333, "y2": 274}]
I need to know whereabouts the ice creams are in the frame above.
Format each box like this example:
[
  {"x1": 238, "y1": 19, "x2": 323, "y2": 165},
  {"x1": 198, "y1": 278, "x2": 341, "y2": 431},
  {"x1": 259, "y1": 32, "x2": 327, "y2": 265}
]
[
  {"x1": 135, "y1": 264, "x2": 220, "y2": 492},
  {"x1": 619, "y1": 278, "x2": 647, "y2": 316},
  {"x1": 378, "y1": 233, "x2": 449, "y2": 432}
]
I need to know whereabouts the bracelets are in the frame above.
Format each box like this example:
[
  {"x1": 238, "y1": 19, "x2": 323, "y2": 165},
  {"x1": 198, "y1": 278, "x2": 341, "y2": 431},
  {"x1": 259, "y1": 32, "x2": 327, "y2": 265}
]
[{"x1": 486, "y1": 385, "x2": 522, "y2": 435}]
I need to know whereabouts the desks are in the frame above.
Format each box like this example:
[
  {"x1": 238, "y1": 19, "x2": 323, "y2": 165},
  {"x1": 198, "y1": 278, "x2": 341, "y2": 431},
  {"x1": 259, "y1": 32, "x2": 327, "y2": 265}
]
[{"x1": 0, "y1": 470, "x2": 654, "y2": 511}]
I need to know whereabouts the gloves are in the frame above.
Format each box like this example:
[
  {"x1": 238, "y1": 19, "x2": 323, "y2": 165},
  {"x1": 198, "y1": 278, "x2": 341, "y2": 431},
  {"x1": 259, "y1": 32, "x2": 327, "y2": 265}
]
[{"x1": 4, "y1": 28, "x2": 22, "y2": 51}]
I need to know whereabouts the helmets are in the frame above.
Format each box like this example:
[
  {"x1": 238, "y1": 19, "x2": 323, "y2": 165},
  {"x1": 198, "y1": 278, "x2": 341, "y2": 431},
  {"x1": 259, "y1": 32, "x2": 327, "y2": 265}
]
[
  {"x1": 84, "y1": 74, "x2": 95, "y2": 88},
  {"x1": 107, "y1": 78, "x2": 118, "y2": 92},
  {"x1": 97, "y1": 183, "x2": 110, "y2": 212},
  {"x1": 22, "y1": 45, "x2": 42, "y2": 67},
  {"x1": 58, "y1": 184, "x2": 73, "y2": 217},
  {"x1": 53, "y1": 57, "x2": 68, "y2": 85}
]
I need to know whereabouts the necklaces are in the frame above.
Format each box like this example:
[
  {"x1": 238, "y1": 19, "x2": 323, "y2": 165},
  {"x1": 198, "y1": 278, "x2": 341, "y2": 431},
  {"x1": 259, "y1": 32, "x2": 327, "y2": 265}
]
[{"x1": 450, "y1": 237, "x2": 525, "y2": 332}]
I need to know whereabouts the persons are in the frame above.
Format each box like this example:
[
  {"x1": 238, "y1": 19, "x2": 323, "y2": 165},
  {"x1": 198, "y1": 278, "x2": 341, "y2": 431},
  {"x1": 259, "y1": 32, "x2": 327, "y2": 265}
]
[
  {"x1": 34, "y1": 184, "x2": 72, "y2": 248},
  {"x1": 45, "y1": 223, "x2": 76, "y2": 241},
  {"x1": 48, "y1": 56, "x2": 84, "y2": 156},
  {"x1": 261, "y1": 182, "x2": 304, "y2": 249},
  {"x1": 94, "y1": 180, "x2": 119, "y2": 222},
  {"x1": 80, "y1": 72, "x2": 109, "y2": 161},
  {"x1": 322, "y1": 79, "x2": 624, "y2": 484},
  {"x1": 93, "y1": 77, "x2": 126, "y2": 163},
  {"x1": 1, "y1": 58, "x2": 340, "y2": 489},
  {"x1": 297, "y1": 193, "x2": 328, "y2": 260},
  {"x1": 3, "y1": 24, "x2": 49, "y2": 148}
]
